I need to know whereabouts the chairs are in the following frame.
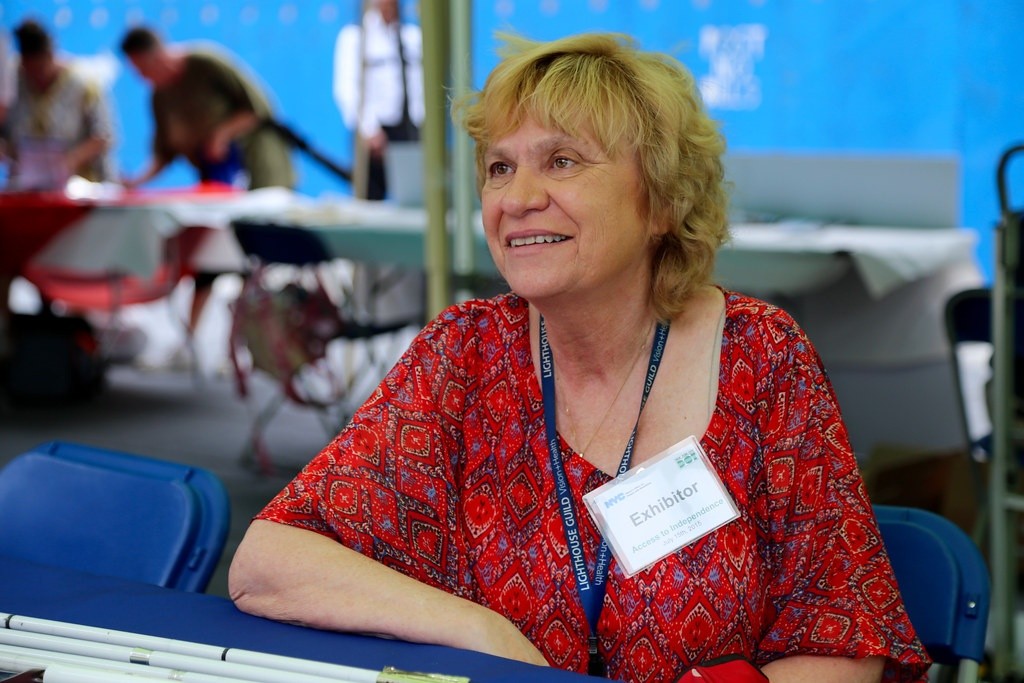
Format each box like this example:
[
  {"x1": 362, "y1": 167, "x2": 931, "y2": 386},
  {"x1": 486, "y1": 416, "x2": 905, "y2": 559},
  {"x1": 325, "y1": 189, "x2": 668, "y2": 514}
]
[
  {"x1": 14, "y1": 198, "x2": 220, "y2": 399},
  {"x1": 1, "y1": 440, "x2": 231, "y2": 597},
  {"x1": 230, "y1": 220, "x2": 426, "y2": 469},
  {"x1": 853, "y1": 501, "x2": 992, "y2": 683}
]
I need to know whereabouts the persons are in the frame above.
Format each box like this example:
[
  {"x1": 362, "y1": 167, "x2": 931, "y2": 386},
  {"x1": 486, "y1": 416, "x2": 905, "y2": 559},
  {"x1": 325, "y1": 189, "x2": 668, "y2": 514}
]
[
  {"x1": 333, "y1": 0, "x2": 425, "y2": 200},
  {"x1": 227, "y1": 26, "x2": 934, "y2": 683},
  {"x1": 1, "y1": 15, "x2": 114, "y2": 192},
  {"x1": 113, "y1": 25, "x2": 295, "y2": 339}
]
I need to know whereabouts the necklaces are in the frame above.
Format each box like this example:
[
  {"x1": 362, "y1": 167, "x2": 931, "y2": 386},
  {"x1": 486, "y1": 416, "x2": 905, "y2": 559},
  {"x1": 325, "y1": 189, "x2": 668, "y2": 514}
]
[{"x1": 554, "y1": 309, "x2": 655, "y2": 459}]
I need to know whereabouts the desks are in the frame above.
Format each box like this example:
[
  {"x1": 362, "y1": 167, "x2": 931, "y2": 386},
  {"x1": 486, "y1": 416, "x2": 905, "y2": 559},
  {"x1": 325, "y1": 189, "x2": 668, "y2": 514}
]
[
  {"x1": 2, "y1": 553, "x2": 620, "y2": 681},
  {"x1": 2, "y1": 180, "x2": 983, "y2": 359}
]
